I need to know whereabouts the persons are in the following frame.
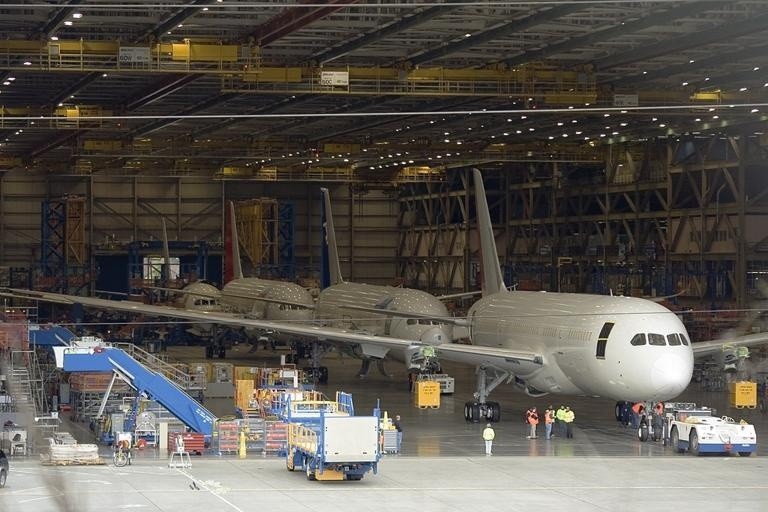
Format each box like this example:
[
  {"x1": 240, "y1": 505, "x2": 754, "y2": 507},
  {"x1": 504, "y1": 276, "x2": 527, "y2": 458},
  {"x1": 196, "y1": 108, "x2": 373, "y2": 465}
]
[
  {"x1": 631, "y1": 403, "x2": 645, "y2": 429},
  {"x1": 483, "y1": 422, "x2": 495, "y2": 455},
  {"x1": 654, "y1": 400, "x2": 664, "y2": 428},
  {"x1": 90, "y1": 420, "x2": 96, "y2": 432},
  {"x1": 395, "y1": 415, "x2": 403, "y2": 455},
  {"x1": 526, "y1": 403, "x2": 576, "y2": 439},
  {"x1": 620, "y1": 398, "x2": 632, "y2": 426}
]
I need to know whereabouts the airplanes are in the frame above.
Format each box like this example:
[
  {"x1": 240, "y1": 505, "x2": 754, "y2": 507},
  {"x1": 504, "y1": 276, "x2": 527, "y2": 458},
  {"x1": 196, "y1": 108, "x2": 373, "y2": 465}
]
[
  {"x1": 40, "y1": 165, "x2": 767, "y2": 426},
  {"x1": 16, "y1": 182, "x2": 519, "y2": 393},
  {"x1": 0, "y1": 216, "x2": 221, "y2": 343},
  {"x1": 0, "y1": 198, "x2": 316, "y2": 368}
]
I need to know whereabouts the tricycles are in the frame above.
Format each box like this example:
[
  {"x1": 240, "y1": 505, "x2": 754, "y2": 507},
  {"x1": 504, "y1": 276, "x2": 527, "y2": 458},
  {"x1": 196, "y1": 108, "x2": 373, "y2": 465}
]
[{"x1": 111, "y1": 440, "x2": 131, "y2": 467}]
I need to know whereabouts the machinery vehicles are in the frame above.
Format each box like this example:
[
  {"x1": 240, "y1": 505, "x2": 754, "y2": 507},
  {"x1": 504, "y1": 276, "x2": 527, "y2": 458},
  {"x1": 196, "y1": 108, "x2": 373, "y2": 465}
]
[
  {"x1": 609, "y1": 397, "x2": 755, "y2": 456},
  {"x1": 276, "y1": 388, "x2": 388, "y2": 482}
]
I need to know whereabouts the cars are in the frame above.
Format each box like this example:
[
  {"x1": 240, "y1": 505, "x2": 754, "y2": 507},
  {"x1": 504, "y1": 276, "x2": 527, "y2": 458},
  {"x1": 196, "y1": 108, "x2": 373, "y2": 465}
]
[{"x1": 0, "y1": 448, "x2": 9, "y2": 488}]
